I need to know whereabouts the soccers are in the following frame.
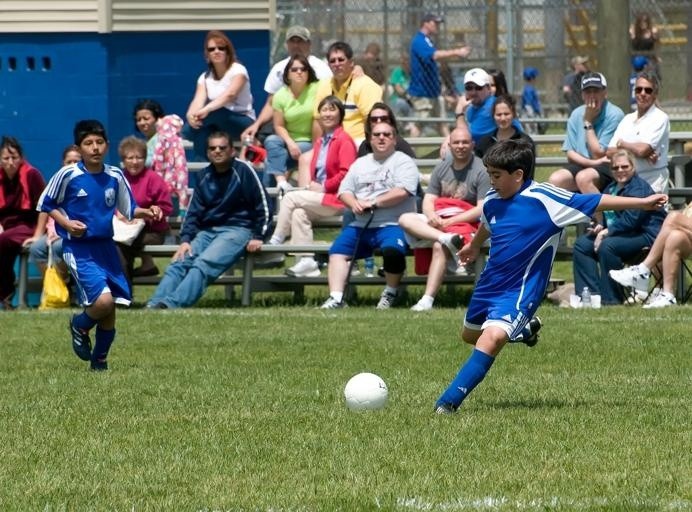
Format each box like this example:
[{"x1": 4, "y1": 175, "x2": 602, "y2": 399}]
[{"x1": 344, "y1": 372, "x2": 389, "y2": 414}]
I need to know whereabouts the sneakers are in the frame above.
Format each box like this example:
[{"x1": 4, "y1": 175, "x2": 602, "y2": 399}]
[
  {"x1": 640, "y1": 292, "x2": 679, "y2": 310},
  {"x1": 374, "y1": 289, "x2": 397, "y2": 308},
  {"x1": 442, "y1": 232, "x2": 463, "y2": 265},
  {"x1": 90, "y1": 355, "x2": 110, "y2": 371},
  {"x1": 409, "y1": 297, "x2": 434, "y2": 312},
  {"x1": 318, "y1": 295, "x2": 349, "y2": 309},
  {"x1": 433, "y1": 403, "x2": 454, "y2": 415},
  {"x1": 522, "y1": 315, "x2": 542, "y2": 347},
  {"x1": 283, "y1": 255, "x2": 323, "y2": 279},
  {"x1": 68, "y1": 312, "x2": 93, "y2": 362},
  {"x1": 604, "y1": 264, "x2": 651, "y2": 293}
]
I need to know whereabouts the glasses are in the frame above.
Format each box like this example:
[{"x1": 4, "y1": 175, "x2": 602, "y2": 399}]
[
  {"x1": 465, "y1": 83, "x2": 488, "y2": 92},
  {"x1": 370, "y1": 115, "x2": 390, "y2": 122},
  {"x1": 609, "y1": 164, "x2": 630, "y2": 171},
  {"x1": 289, "y1": 66, "x2": 309, "y2": 73},
  {"x1": 634, "y1": 86, "x2": 654, "y2": 96},
  {"x1": 209, "y1": 145, "x2": 229, "y2": 151},
  {"x1": 329, "y1": 57, "x2": 346, "y2": 63},
  {"x1": 207, "y1": 46, "x2": 228, "y2": 52},
  {"x1": 372, "y1": 132, "x2": 394, "y2": 137},
  {"x1": 64, "y1": 159, "x2": 79, "y2": 164}
]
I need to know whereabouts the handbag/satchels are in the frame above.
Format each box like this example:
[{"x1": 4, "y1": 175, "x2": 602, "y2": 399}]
[{"x1": 37, "y1": 241, "x2": 72, "y2": 309}]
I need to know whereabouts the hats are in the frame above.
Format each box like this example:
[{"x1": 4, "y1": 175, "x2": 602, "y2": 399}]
[
  {"x1": 522, "y1": 66, "x2": 539, "y2": 80},
  {"x1": 579, "y1": 71, "x2": 608, "y2": 91},
  {"x1": 463, "y1": 67, "x2": 490, "y2": 87},
  {"x1": 284, "y1": 25, "x2": 313, "y2": 42},
  {"x1": 631, "y1": 55, "x2": 650, "y2": 70},
  {"x1": 421, "y1": 13, "x2": 445, "y2": 24}
]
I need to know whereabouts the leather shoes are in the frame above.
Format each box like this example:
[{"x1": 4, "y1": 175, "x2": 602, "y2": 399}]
[{"x1": 133, "y1": 264, "x2": 160, "y2": 279}]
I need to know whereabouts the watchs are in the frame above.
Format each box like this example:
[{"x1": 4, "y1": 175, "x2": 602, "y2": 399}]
[{"x1": 370, "y1": 199, "x2": 374, "y2": 208}]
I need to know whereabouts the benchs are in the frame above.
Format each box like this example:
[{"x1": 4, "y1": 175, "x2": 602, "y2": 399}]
[
  {"x1": 16, "y1": 242, "x2": 689, "y2": 308},
  {"x1": 394, "y1": 116, "x2": 691, "y2": 123},
  {"x1": 183, "y1": 187, "x2": 692, "y2": 212},
  {"x1": 181, "y1": 131, "x2": 692, "y2": 149},
  {"x1": 118, "y1": 154, "x2": 691, "y2": 187},
  {"x1": 163, "y1": 214, "x2": 594, "y2": 242}
]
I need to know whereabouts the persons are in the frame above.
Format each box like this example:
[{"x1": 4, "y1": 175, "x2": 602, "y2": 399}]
[
  {"x1": 606, "y1": 72, "x2": 670, "y2": 205},
  {"x1": 487, "y1": 67, "x2": 516, "y2": 113},
  {"x1": 143, "y1": 132, "x2": 270, "y2": 309},
  {"x1": 265, "y1": 55, "x2": 326, "y2": 191},
  {"x1": 630, "y1": 12, "x2": 658, "y2": 58},
  {"x1": 442, "y1": 69, "x2": 523, "y2": 163},
  {"x1": 631, "y1": 54, "x2": 660, "y2": 111},
  {"x1": 255, "y1": 95, "x2": 357, "y2": 275},
  {"x1": 436, "y1": 141, "x2": 668, "y2": 416},
  {"x1": 31, "y1": 144, "x2": 82, "y2": 287},
  {"x1": 390, "y1": 52, "x2": 417, "y2": 134},
  {"x1": 1, "y1": 137, "x2": 47, "y2": 308},
  {"x1": 310, "y1": 41, "x2": 384, "y2": 153},
  {"x1": 550, "y1": 74, "x2": 625, "y2": 228},
  {"x1": 241, "y1": 27, "x2": 332, "y2": 148},
  {"x1": 570, "y1": 152, "x2": 668, "y2": 308},
  {"x1": 356, "y1": 100, "x2": 418, "y2": 157},
  {"x1": 520, "y1": 64, "x2": 543, "y2": 135},
  {"x1": 317, "y1": 124, "x2": 419, "y2": 311},
  {"x1": 23, "y1": 119, "x2": 136, "y2": 369},
  {"x1": 608, "y1": 201, "x2": 692, "y2": 309},
  {"x1": 407, "y1": 16, "x2": 471, "y2": 135},
  {"x1": 184, "y1": 32, "x2": 256, "y2": 161},
  {"x1": 113, "y1": 136, "x2": 173, "y2": 283},
  {"x1": 133, "y1": 100, "x2": 189, "y2": 281},
  {"x1": 472, "y1": 94, "x2": 535, "y2": 182},
  {"x1": 358, "y1": 43, "x2": 383, "y2": 85},
  {"x1": 399, "y1": 129, "x2": 493, "y2": 314},
  {"x1": 563, "y1": 55, "x2": 594, "y2": 114}
]
